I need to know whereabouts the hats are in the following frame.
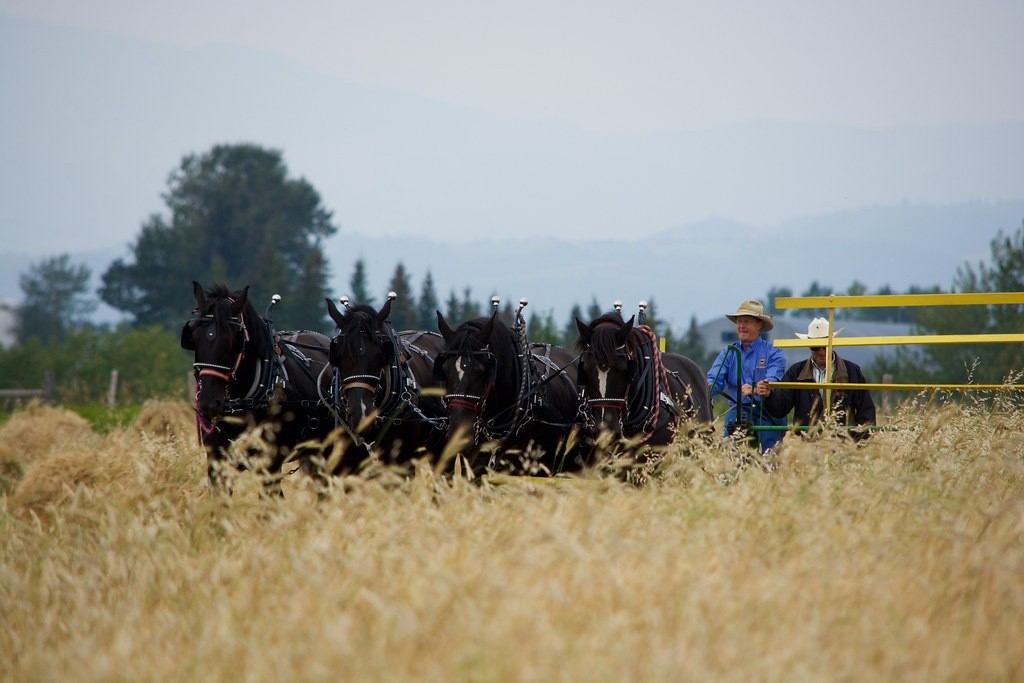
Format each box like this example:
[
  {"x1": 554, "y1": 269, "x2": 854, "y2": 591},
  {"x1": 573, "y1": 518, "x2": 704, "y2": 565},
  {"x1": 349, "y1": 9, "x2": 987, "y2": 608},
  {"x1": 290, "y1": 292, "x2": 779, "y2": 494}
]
[
  {"x1": 725, "y1": 301, "x2": 774, "y2": 332},
  {"x1": 795, "y1": 317, "x2": 844, "y2": 340}
]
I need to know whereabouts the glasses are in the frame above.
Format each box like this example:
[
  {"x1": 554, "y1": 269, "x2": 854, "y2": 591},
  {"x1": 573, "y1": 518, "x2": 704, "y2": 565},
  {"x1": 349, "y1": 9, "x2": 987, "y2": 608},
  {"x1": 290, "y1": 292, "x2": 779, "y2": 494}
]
[{"x1": 810, "y1": 347, "x2": 827, "y2": 351}]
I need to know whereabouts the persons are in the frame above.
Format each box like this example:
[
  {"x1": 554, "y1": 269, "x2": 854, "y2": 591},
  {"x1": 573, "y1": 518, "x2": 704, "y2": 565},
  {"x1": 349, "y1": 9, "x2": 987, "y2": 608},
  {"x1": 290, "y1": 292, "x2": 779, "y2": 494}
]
[
  {"x1": 757, "y1": 317, "x2": 876, "y2": 443},
  {"x1": 705, "y1": 300, "x2": 787, "y2": 455}
]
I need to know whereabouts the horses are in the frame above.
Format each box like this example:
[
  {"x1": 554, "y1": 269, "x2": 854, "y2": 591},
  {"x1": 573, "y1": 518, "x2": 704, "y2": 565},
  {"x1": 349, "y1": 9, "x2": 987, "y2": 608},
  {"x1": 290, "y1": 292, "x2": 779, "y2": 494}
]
[{"x1": 181, "y1": 278, "x2": 714, "y2": 504}]
